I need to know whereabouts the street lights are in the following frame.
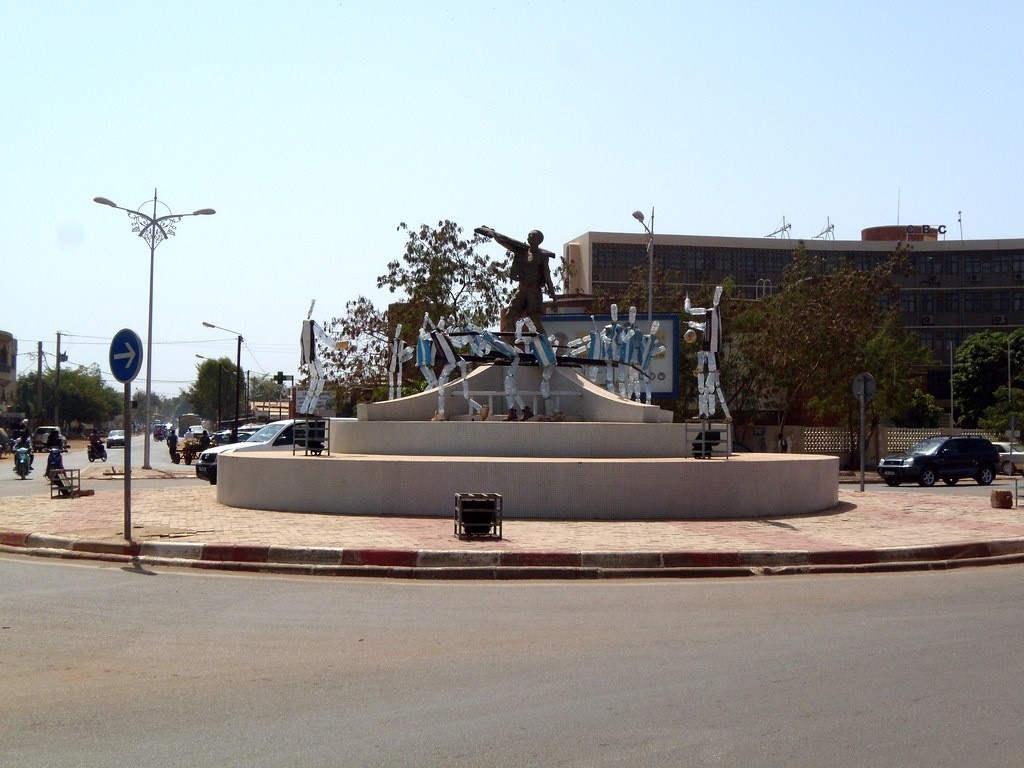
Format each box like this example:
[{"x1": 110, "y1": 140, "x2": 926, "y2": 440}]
[
  {"x1": 94, "y1": 197, "x2": 217, "y2": 470},
  {"x1": 196, "y1": 355, "x2": 222, "y2": 429},
  {"x1": 203, "y1": 322, "x2": 242, "y2": 442},
  {"x1": 632, "y1": 211, "x2": 654, "y2": 334}
]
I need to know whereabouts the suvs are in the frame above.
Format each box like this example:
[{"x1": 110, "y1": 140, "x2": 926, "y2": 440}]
[
  {"x1": 31, "y1": 425, "x2": 68, "y2": 452},
  {"x1": 195, "y1": 418, "x2": 358, "y2": 485},
  {"x1": 876, "y1": 434, "x2": 1001, "y2": 487}
]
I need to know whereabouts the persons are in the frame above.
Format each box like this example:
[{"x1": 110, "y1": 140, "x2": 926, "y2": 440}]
[
  {"x1": 13, "y1": 434, "x2": 34, "y2": 471},
  {"x1": 229, "y1": 430, "x2": 238, "y2": 443},
  {"x1": 200, "y1": 430, "x2": 210, "y2": 449},
  {"x1": 166, "y1": 430, "x2": 179, "y2": 463},
  {"x1": 157, "y1": 426, "x2": 166, "y2": 438},
  {"x1": 185, "y1": 427, "x2": 194, "y2": 440},
  {"x1": 483, "y1": 226, "x2": 558, "y2": 366},
  {"x1": 47, "y1": 428, "x2": 64, "y2": 448},
  {"x1": 137, "y1": 423, "x2": 143, "y2": 431},
  {"x1": 90, "y1": 427, "x2": 106, "y2": 454}
]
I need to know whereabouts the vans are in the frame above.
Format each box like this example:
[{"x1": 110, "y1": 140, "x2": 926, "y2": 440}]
[{"x1": 153, "y1": 424, "x2": 167, "y2": 433}]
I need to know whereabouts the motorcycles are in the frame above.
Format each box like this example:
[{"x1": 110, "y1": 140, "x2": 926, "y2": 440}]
[
  {"x1": 44, "y1": 445, "x2": 68, "y2": 483},
  {"x1": 154, "y1": 433, "x2": 167, "y2": 443},
  {"x1": 13, "y1": 447, "x2": 31, "y2": 479},
  {"x1": 86, "y1": 440, "x2": 107, "y2": 462}
]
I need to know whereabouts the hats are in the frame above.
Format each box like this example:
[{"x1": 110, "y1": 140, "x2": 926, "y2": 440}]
[
  {"x1": 172, "y1": 430, "x2": 175, "y2": 434},
  {"x1": 203, "y1": 430, "x2": 208, "y2": 435}
]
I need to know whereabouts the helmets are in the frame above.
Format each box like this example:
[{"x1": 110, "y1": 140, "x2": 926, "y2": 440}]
[
  {"x1": 92, "y1": 428, "x2": 97, "y2": 433},
  {"x1": 50, "y1": 428, "x2": 59, "y2": 435}
]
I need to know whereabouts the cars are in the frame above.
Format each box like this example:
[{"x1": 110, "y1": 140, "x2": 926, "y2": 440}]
[
  {"x1": 211, "y1": 428, "x2": 255, "y2": 443},
  {"x1": 107, "y1": 429, "x2": 125, "y2": 448},
  {"x1": 992, "y1": 442, "x2": 1024, "y2": 477},
  {"x1": 188, "y1": 426, "x2": 205, "y2": 435}
]
